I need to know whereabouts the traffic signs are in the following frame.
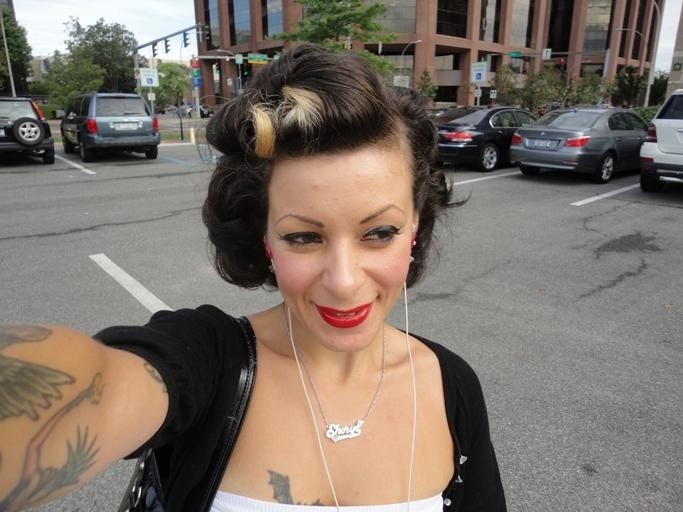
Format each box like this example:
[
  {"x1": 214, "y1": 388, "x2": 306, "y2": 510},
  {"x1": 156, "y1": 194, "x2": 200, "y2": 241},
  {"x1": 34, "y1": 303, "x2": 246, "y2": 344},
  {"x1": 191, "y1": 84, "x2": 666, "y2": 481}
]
[
  {"x1": 247, "y1": 53, "x2": 268, "y2": 65},
  {"x1": 470, "y1": 62, "x2": 488, "y2": 84},
  {"x1": 508, "y1": 50, "x2": 521, "y2": 59}
]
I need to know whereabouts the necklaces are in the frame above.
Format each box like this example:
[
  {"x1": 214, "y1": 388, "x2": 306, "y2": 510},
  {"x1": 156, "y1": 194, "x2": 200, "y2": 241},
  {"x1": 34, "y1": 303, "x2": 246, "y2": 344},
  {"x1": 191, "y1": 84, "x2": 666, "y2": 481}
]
[{"x1": 282, "y1": 301, "x2": 386, "y2": 442}]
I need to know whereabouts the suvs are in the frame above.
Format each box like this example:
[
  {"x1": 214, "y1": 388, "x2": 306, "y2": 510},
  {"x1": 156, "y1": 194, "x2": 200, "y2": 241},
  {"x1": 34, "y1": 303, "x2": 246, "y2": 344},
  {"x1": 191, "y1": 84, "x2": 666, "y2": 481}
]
[
  {"x1": 639, "y1": 90, "x2": 683, "y2": 193},
  {"x1": 0, "y1": 97, "x2": 54, "y2": 165},
  {"x1": 60, "y1": 91, "x2": 160, "y2": 162}
]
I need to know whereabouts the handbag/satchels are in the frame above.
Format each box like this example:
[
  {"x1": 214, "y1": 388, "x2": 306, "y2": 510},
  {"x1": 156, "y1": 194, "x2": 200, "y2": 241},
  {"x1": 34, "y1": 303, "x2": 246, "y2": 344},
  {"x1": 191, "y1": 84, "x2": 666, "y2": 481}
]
[{"x1": 118, "y1": 445, "x2": 167, "y2": 512}]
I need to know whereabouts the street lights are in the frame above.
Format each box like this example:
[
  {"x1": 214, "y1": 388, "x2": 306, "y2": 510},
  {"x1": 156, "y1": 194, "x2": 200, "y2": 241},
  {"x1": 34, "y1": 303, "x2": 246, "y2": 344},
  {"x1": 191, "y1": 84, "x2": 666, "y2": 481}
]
[
  {"x1": 398, "y1": 40, "x2": 422, "y2": 74},
  {"x1": 217, "y1": 49, "x2": 242, "y2": 90}
]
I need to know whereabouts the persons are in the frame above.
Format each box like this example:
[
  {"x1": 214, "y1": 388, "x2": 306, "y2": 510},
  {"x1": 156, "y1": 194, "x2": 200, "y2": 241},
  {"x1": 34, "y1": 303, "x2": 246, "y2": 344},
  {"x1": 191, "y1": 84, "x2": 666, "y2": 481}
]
[{"x1": 0, "y1": 46, "x2": 510, "y2": 512}]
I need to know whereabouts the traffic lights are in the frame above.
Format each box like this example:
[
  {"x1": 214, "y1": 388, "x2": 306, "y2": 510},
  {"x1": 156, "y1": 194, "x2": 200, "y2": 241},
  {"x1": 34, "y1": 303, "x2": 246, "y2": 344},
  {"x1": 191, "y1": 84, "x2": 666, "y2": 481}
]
[
  {"x1": 203, "y1": 24, "x2": 210, "y2": 41},
  {"x1": 152, "y1": 43, "x2": 158, "y2": 57},
  {"x1": 560, "y1": 57, "x2": 565, "y2": 71},
  {"x1": 183, "y1": 32, "x2": 190, "y2": 47},
  {"x1": 164, "y1": 38, "x2": 171, "y2": 53}
]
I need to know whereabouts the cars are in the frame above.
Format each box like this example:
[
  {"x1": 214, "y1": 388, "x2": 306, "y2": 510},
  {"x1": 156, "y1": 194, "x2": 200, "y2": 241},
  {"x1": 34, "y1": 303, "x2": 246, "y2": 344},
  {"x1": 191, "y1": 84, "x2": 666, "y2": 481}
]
[
  {"x1": 511, "y1": 104, "x2": 649, "y2": 184},
  {"x1": 430, "y1": 106, "x2": 538, "y2": 172}
]
